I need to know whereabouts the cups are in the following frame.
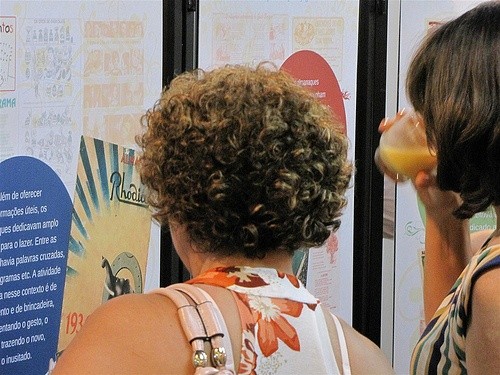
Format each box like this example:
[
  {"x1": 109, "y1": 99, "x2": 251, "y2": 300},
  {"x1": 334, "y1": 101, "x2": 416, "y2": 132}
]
[{"x1": 379, "y1": 103, "x2": 437, "y2": 181}]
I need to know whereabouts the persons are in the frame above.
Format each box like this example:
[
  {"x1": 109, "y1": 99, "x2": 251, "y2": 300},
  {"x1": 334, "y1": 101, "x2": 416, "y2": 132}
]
[
  {"x1": 50, "y1": 64, "x2": 399, "y2": 375},
  {"x1": 375, "y1": 0, "x2": 500, "y2": 375}
]
